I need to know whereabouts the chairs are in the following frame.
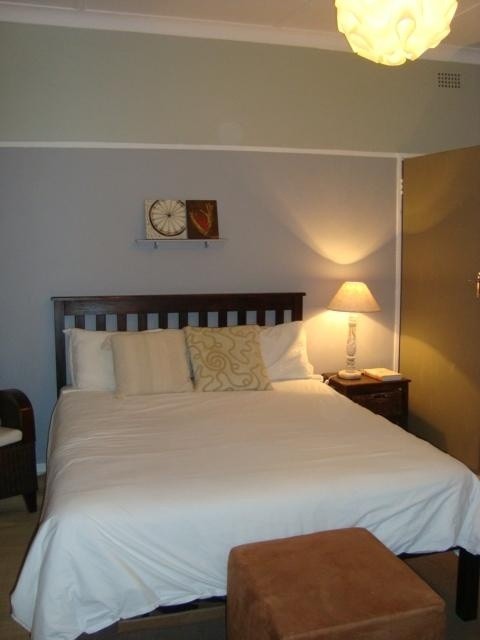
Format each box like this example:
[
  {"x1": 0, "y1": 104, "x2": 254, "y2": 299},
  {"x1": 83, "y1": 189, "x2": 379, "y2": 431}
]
[{"x1": 0, "y1": 389, "x2": 38, "y2": 516}]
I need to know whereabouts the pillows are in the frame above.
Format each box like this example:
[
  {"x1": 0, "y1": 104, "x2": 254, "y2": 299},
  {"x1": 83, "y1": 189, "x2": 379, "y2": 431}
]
[
  {"x1": 186, "y1": 325, "x2": 268, "y2": 390},
  {"x1": 112, "y1": 329, "x2": 187, "y2": 395},
  {"x1": 63, "y1": 327, "x2": 167, "y2": 389},
  {"x1": 263, "y1": 321, "x2": 309, "y2": 380}
]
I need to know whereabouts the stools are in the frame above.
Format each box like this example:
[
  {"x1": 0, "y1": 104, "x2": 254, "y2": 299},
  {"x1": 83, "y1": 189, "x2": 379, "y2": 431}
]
[{"x1": 225, "y1": 528, "x2": 446, "y2": 640}]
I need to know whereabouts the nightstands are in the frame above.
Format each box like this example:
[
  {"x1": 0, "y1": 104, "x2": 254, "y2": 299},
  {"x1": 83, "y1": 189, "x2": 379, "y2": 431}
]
[
  {"x1": 323, "y1": 374, "x2": 410, "y2": 430},
  {"x1": 8, "y1": 293, "x2": 480, "y2": 640}
]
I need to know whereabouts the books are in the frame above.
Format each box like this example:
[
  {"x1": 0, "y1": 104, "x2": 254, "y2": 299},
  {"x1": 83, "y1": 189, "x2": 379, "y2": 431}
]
[{"x1": 361, "y1": 367, "x2": 403, "y2": 382}]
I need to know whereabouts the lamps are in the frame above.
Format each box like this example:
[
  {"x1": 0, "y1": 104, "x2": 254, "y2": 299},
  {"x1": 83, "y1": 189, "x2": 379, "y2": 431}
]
[
  {"x1": 335, "y1": 0, "x2": 459, "y2": 67},
  {"x1": 326, "y1": 282, "x2": 381, "y2": 379}
]
